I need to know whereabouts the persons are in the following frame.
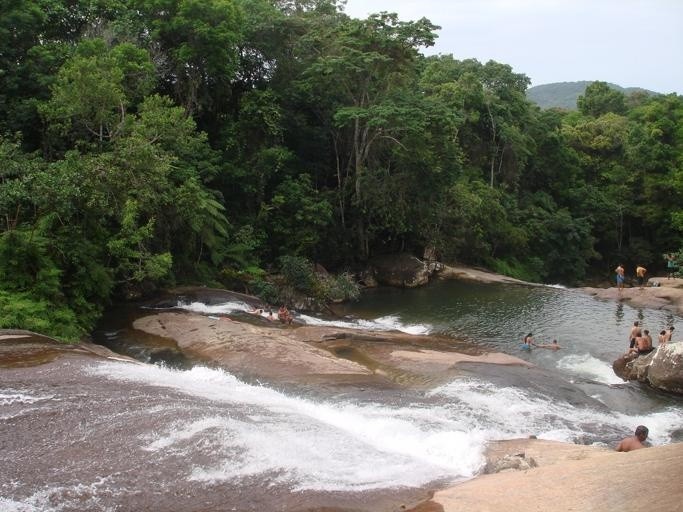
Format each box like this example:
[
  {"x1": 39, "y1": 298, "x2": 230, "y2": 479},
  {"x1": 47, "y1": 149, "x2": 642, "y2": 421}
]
[
  {"x1": 658, "y1": 331, "x2": 666, "y2": 342},
  {"x1": 641, "y1": 330, "x2": 652, "y2": 353},
  {"x1": 628, "y1": 320, "x2": 642, "y2": 348},
  {"x1": 615, "y1": 264, "x2": 625, "y2": 292},
  {"x1": 628, "y1": 333, "x2": 648, "y2": 355},
  {"x1": 545, "y1": 339, "x2": 561, "y2": 350},
  {"x1": 666, "y1": 253, "x2": 676, "y2": 280},
  {"x1": 636, "y1": 263, "x2": 647, "y2": 290},
  {"x1": 614, "y1": 425, "x2": 649, "y2": 452},
  {"x1": 247, "y1": 303, "x2": 293, "y2": 325},
  {"x1": 520, "y1": 332, "x2": 537, "y2": 352},
  {"x1": 666, "y1": 327, "x2": 675, "y2": 341}
]
